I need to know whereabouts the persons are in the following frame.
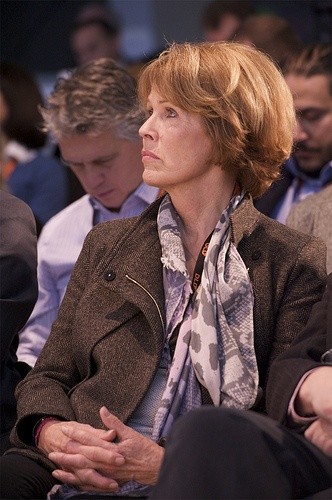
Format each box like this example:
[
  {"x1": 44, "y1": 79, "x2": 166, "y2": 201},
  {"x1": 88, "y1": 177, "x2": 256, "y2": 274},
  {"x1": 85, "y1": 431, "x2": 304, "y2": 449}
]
[
  {"x1": 0, "y1": 1, "x2": 303, "y2": 239},
  {"x1": 150, "y1": 271, "x2": 332, "y2": 500},
  {"x1": 0, "y1": 192, "x2": 40, "y2": 381},
  {"x1": 10, "y1": 57, "x2": 160, "y2": 379},
  {"x1": 253, "y1": 39, "x2": 332, "y2": 225},
  {"x1": 0, "y1": 40, "x2": 327, "y2": 500},
  {"x1": 285, "y1": 182, "x2": 331, "y2": 274}
]
